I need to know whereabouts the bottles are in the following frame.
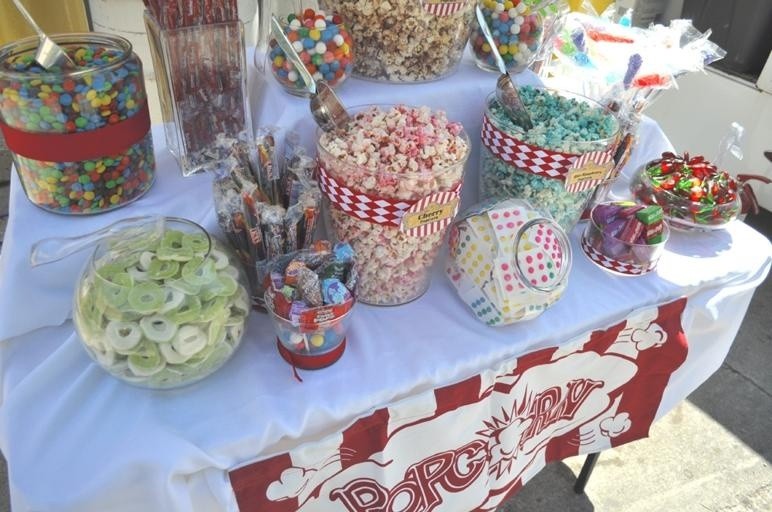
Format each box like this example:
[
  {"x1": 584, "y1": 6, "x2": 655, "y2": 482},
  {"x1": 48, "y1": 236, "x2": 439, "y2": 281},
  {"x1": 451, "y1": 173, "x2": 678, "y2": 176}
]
[
  {"x1": 71, "y1": 215, "x2": 250, "y2": 391},
  {"x1": 443, "y1": 193, "x2": 574, "y2": 328}
]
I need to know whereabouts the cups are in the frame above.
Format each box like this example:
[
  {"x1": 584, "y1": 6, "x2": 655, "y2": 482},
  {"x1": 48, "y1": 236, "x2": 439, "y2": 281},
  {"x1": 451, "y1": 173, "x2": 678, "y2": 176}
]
[
  {"x1": 265, "y1": 288, "x2": 357, "y2": 370},
  {"x1": 475, "y1": 87, "x2": 622, "y2": 241},
  {"x1": 315, "y1": 0, "x2": 478, "y2": 84},
  {"x1": 581, "y1": 202, "x2": 672, "y2": 276},
  {"x1": 266, "y1": 10, "x2": 355, "y2": 97},
  {"x1": 237, "y1": 256, "x2": 270, "y2": 316},
  {"x1": 1, "y1": 31, "x2": 156, "y2": 214},
  {"x1": 315, "y1": 103, "x2": 471, "y2": 309},
  {"x1": 472, "y1": 0, "x2": 556, "y2": 76}
]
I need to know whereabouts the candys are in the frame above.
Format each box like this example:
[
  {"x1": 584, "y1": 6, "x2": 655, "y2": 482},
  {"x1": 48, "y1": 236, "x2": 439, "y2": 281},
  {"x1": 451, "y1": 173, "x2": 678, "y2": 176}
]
[
  {"x1": 1, "y1": 0, "x2": 157, "y2": 216},
  {"x1": 208, "y1": 0, "x2": 745, "y2": 390},
  {"x1": 143, "y1": 2, "x2": 257, "y2": 176},
  {"x1": 71, "y1": 219, "x2": 251, "y2": 389}
]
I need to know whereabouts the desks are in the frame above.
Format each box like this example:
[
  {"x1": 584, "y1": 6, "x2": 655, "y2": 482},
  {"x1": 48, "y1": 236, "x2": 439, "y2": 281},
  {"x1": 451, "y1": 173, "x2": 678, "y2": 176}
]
[{"x1": 0, "y1": 43, "x2": 772, "y2": 509}]
